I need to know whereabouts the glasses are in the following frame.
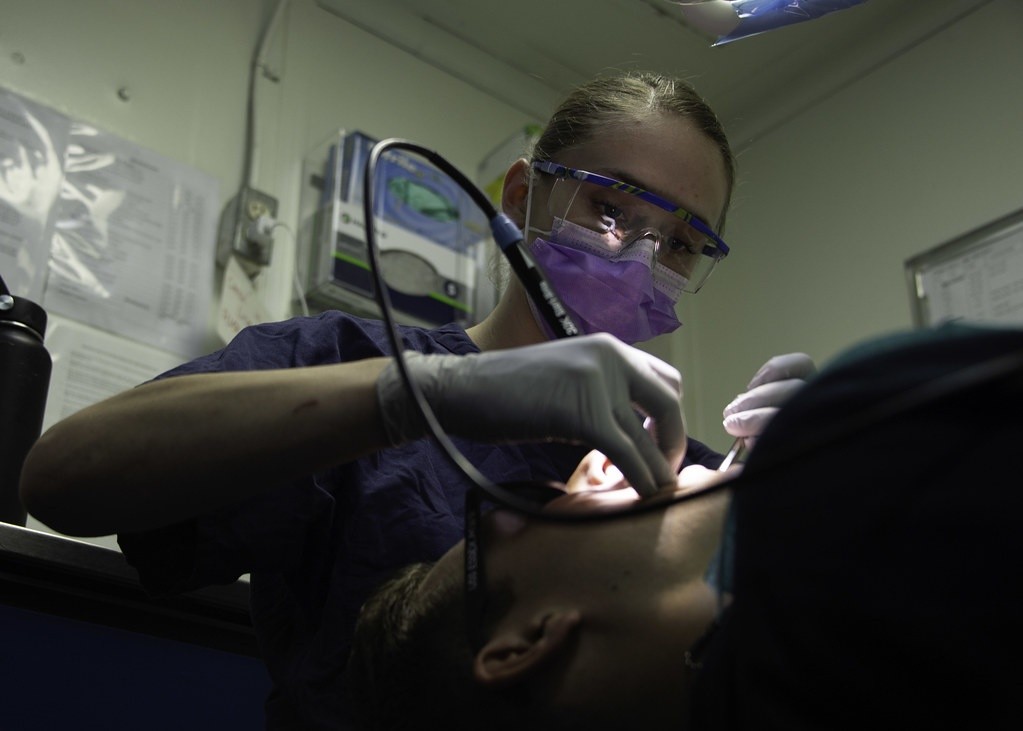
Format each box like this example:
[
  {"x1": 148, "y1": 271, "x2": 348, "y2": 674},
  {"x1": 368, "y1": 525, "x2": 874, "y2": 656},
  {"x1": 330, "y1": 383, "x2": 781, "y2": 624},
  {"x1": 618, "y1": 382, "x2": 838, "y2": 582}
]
[{"x1": 532, "y1": 160, "x2": 730, "y2": 294}]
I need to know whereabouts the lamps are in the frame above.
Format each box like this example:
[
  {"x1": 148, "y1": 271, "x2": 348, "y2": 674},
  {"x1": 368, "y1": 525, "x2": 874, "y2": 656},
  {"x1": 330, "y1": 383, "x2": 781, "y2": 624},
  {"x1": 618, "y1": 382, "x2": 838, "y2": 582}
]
[{"x1": 710, "y1": 0, "x2": 865, "y2": 47}]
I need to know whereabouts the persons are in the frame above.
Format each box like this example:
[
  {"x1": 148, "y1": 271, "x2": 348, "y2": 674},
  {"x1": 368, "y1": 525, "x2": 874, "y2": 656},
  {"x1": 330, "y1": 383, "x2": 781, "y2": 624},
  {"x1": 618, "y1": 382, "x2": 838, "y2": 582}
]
[
  {"x1": 19, "y1": 72, "x2": 1023, "y2": 731},
  {"x1": 352, "y1": 317, "x2": 1023, "y2": 730}
]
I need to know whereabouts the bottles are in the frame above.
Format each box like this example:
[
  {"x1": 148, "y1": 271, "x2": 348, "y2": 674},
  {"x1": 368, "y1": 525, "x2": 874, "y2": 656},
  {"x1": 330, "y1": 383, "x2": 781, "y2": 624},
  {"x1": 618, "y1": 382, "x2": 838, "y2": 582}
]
[{"x1": 0, "y1": 275, "x2": 53, "y2": 526}]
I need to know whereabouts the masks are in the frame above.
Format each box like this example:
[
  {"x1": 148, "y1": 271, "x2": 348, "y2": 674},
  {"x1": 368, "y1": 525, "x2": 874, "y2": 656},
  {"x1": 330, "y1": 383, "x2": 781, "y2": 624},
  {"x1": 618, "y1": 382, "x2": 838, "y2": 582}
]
[{"x1": 520, "y1": 171, "x2": 689, "y2": 345}]
